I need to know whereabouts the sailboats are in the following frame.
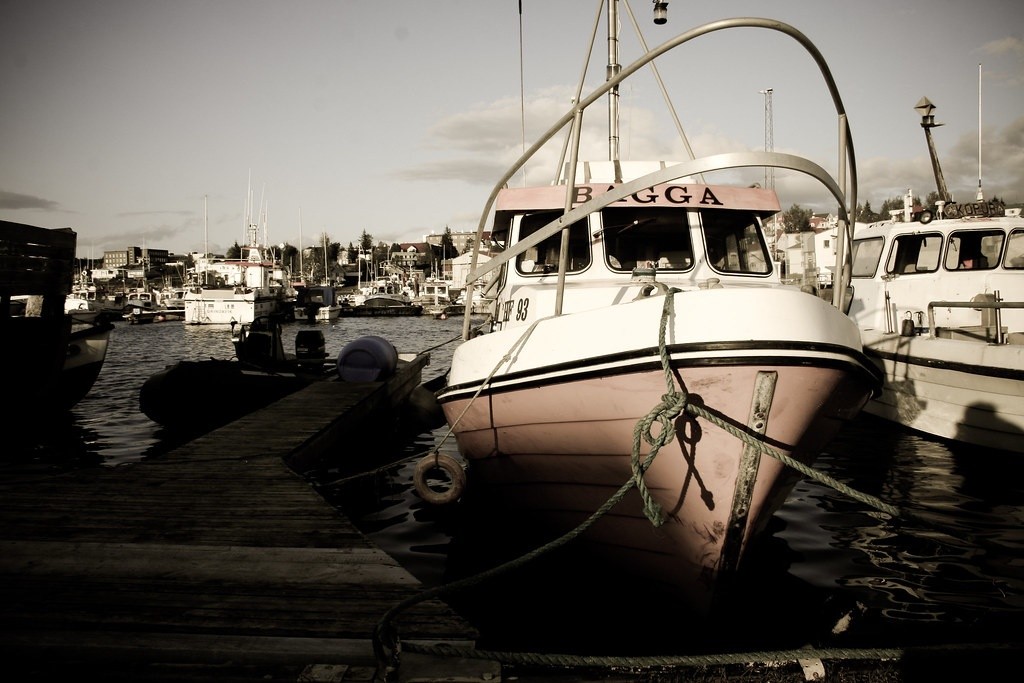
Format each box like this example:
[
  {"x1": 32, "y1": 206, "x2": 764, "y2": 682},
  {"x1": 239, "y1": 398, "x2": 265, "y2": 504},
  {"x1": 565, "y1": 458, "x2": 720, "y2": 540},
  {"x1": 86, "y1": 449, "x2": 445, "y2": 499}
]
[{"x1": 65, "y1": 168, "x2": 449, "y2": 326}]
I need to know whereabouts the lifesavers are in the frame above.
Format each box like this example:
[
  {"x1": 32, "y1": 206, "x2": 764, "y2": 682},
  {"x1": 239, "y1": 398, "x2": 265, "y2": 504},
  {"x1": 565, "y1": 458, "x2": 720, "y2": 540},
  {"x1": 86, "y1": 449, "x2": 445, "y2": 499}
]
[{"x1": 412, "y1": 450, "x2": 469, "y2": 505}]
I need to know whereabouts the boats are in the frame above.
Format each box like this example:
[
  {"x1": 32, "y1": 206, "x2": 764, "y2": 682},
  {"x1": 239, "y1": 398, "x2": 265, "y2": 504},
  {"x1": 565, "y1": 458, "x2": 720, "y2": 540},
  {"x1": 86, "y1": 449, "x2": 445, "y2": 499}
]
[
  {"x1": 432, "y1": 0, "x2": 888, "y2": 654},
  {"x1": 843, "y1": 62, "x2": 1024, "y2": 453},
  {"x1": 139, "y1": 316, "x2": 339, "y2": 427}
]
